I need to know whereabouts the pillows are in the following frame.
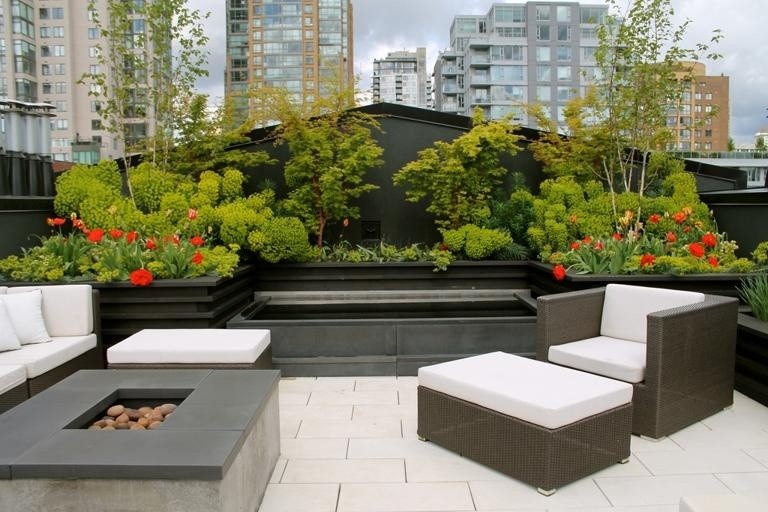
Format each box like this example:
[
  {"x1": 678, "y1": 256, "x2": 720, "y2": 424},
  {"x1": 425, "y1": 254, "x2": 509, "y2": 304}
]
[
  {"x1": 1, "y1": 290, "x2": 51, "y2": 345},
  {"x1": 0, "y1": 296, "x2": 20, "y2": 352}
]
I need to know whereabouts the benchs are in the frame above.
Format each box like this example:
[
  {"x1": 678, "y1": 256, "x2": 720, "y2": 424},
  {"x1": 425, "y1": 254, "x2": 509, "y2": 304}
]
[
  {"x1": 417, "y1": 351, "x2": 632, "y2": 495},
  {"x1": 106, "y1": 328, "x2": 272, "y2": 370}
]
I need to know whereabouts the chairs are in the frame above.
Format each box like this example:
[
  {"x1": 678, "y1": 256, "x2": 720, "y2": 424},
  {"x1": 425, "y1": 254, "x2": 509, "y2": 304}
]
[{"x1": 536, "y1": 283, "x2": 739, "y2": 441}]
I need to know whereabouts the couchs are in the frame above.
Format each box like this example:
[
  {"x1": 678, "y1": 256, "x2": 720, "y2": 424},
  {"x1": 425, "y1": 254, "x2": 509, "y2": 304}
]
[{"x1": 0, "y1": 284, "x2": 102, "y2": 413}]
